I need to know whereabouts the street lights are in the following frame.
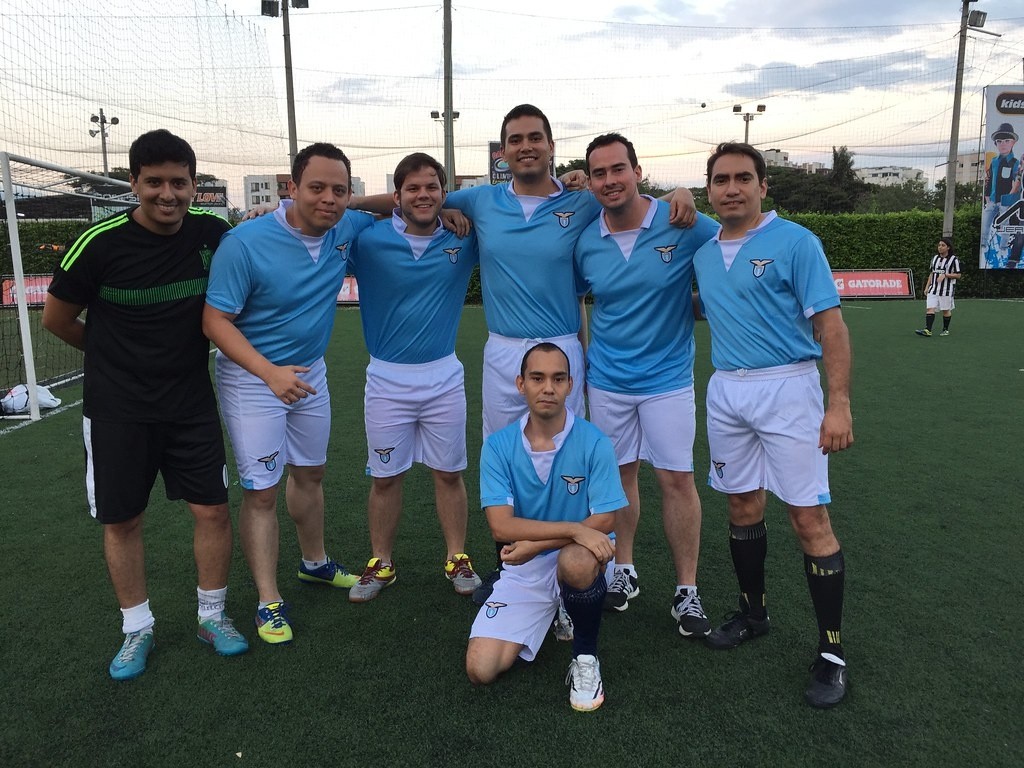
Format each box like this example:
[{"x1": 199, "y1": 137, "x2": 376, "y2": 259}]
[
  {"x1": 89, "y1": 108, "x2": 119, "y2": 178},
  {"x1": 733, "y1": 104, "x2": 766, "y2": 145},
  {"x1": 942, "y1": 10, "x2": 987, "y2": 238},
  {"x1": 261, "y1": 0, "x2": 308, "y2": 175}
]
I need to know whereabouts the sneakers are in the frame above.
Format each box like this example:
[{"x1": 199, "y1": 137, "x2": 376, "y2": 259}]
[
  {"x1": 472, "y1": 566, "x2": 502, "y2": 606},
  {"x1": 671, "y1": 588, "x2": 711, "y2": 638},
  {"x1": 565, "y1": 654, "x2": 604, "y2": 711},
  {"x1": 940, "y1": 330, "x2": 949, "y2": 336},
  {"x1": 298, "y1": 555, "x2": 362, "y2": 589},
  {"x1": 804, "y1": 653, "x2": 846, "y2": 709},
  {"x1": 255, "y1": 601, "x2": 299, "y2": 645},
  {"x1": 915, "y1": 329, "x2": 933, "y2": 337},
  {"x1": 197, "y1": 611, "x2": 248, "y2": 657},
  {"x1": 109, "y1": 629, "x2": 155, "y2": 680},
  {"x1": 349, "y1": 558, "x2": 396, "y2": 603},
  {"x1": 704, "y1": 595, "x2": 771, "y2": 650},
  {"x1": 603, "y1": 568, "x2": 640, "y2": 612},
  {"x1": 552, "y1": 594, "x2": 574, "y2": 642},
  {"x1": 445, "y1": 554, "x2": 482, "y2": 595}
]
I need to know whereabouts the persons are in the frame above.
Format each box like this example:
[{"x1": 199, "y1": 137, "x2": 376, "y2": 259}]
[
  {"x1": 693, "y1": 142, "x2": 849, "y2": 708},
  {"x1": 572, "y1": 133, "x2": 821, "y2": 640},
  {"x1": 978, "y1": 123, "x2": 1024, "y2": 268},
  {"x1": 202, "y1": 142, "x2": 362, "y2": 644},
  {"x1": 242, "y1": 101, "x2": 695, "y2": 605},
  {"x1": 463, "y1": 342, "x2": 630, "y2": 714},
  {"x1": 914, "y1": 236, "x2": 963, "y2": 336},
  {"x1": 41, "y1": 128, "x2": 276, "y2": 680}
]
